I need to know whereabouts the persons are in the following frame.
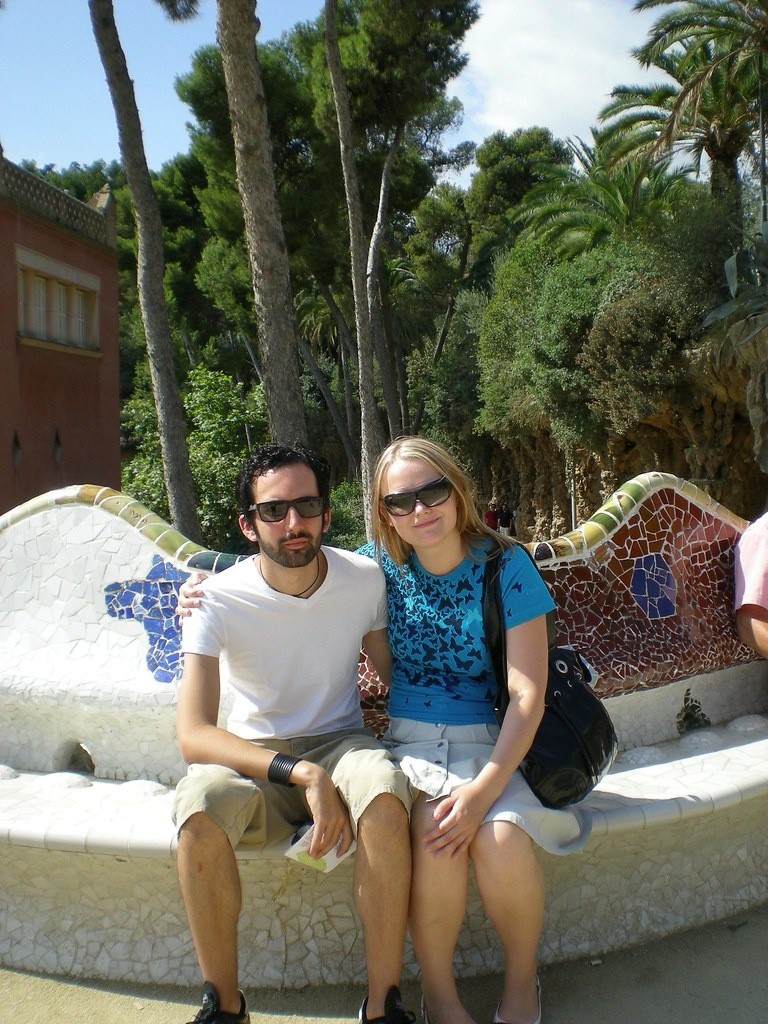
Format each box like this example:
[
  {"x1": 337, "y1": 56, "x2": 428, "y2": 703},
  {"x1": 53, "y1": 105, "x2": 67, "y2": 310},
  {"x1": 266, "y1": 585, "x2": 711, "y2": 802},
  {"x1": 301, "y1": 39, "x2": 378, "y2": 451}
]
[
  {"x1": 172, "y1": 442, "x2": 417, "y2": 1024},
  {"x1": 484, "y1": 505, "x2": 518, "y2": 536},
  {"x1": 176, "y1": 439, "x2": 588, "y2": 1023},
  {"x1": 734, "y1": 511, "x2": 768, "y2": 659}
]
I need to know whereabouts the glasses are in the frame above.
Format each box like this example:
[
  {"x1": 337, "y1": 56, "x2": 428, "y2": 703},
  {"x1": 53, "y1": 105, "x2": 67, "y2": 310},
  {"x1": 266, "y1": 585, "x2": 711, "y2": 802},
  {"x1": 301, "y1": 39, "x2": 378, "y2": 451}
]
[
  {"x1": 382, "y1": 476, "x2": 452, "y2": 516},
  {"x1": 245, "y1": 496, "x2": 327, "y2": 522}
]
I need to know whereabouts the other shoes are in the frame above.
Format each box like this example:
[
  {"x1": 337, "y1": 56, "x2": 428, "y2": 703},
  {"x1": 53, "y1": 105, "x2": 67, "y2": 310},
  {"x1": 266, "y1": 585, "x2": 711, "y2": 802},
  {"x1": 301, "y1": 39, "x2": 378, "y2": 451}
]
[
  {"x1": 186, "y1": 981, "x2": 250, "y2": 1024},
  {"x1": 359, "y1": 986, "x2": 416, "y2": 1024},
  {"x1": 421, "y1": 995, "x2": 429, "y2": 1024},
  {"x1": 492, "y1": 975, "x2": 541, "y2": 1024}
]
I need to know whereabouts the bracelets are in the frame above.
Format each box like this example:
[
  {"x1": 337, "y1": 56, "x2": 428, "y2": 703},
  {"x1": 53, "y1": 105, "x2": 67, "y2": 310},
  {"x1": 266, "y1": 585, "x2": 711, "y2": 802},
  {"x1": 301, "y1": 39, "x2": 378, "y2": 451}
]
[{"x1": 268, "y1": 753, "x2": 302, "y2": 788}]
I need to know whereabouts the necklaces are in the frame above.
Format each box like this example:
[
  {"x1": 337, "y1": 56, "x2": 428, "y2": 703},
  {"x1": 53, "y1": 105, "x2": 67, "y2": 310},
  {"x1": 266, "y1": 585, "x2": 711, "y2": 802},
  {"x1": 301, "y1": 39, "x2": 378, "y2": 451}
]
[{"x1": 260, "y1": 554, "x2": 319, "y2": 596}]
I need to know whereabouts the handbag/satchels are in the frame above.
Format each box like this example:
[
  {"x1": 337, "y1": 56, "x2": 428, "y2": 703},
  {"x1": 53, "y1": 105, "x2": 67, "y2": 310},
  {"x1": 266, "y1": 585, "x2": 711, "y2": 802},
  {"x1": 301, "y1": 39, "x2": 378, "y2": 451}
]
[{"x1": 482, "y1": 540, "x2": 619, "y2": 811}]
